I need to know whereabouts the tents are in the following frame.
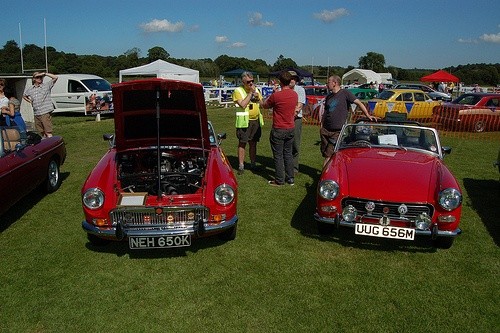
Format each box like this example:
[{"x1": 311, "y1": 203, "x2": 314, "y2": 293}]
[
  {"x1": 342, "y1": 69, "x2": 383, "y2": 86},
  {"x1": 119, "y1": 59, "x2": 199, "y2": 84},
  {"x1": 270, "y1": 67, "x2": 315, "y2": 86},
  {"x1": 221, "y1": 69, "x2": 256, "y2": 85}
]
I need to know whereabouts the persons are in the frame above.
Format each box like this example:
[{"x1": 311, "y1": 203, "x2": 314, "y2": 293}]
[
  {"x1": 433, "y1": 81, "x2": 454, "y2": 93},
  {"x1": 87, "y1": 93, "x2": 111, "y2": 110},
  {"x1": 0, "y1": 79, "x2": 9, "y2": 127},
  {"x1": 23, "y1": 71, "x2": 58, "y2": 139},
  {"x1": 256, "y1": 71, "x2": 299, "y2": 185},
  {"x1": 274, "y1": 70, "x2": 306, "y2": 173},
  {"x1": 474, "y1": 83, "x2": 482, "y2": 93},
  {"x1": 232, "y1": 71, "x2": 264, "y2": 175},
  {"x1": 1, "y1": 84, "x2": 26, "y2": 137},
  {"x1": 493, "y1": 85, "x2": 500, "y2": 92},
  {"x1": 320, "y1": 73, "x2": 381, "y2": 170}
]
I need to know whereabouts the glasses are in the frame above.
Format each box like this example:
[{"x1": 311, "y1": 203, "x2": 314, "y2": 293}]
[
  {"x1": 36, "y1": 76, "x2": 43, "y2": 79},
  {"x1": 247, "y1": 80, "x2": 254, "y2": 84}
]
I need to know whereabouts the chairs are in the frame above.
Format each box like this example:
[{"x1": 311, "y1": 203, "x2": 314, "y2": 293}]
[
  {"x1": 354, "y1": 134, "x2": 370, "y2": 142},
  {"x1": 1, "y1": 129, "x2": 20, "y2": 151},
  {"x1": 404, "y1": 137, "x2": 419, "y2": 145}
]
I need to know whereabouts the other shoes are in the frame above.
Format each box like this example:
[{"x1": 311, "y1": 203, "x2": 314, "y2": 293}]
[
  {"x1": 288, "y1": 181, "x2": 295, "y2": 186},
  {"x1": 268, "y1": 180, "x2": 283, "y2": 187},
  {"x1": 237, "y1": 168, "x2": 244, "y2": 176}
]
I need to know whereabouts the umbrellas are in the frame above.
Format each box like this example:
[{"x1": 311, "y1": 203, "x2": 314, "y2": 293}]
[{"x1": 421, "y1": 70, "x2": 460, "y2": 82}]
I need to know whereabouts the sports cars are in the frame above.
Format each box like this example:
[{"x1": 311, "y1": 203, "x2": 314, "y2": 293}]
[
  {"x1": 79, "y1": 78, "x2": 239, "y2": 250},
  {"x1": 0, "y1": 125, "x2": 67, "y2": 217},
  {"x1": 313, "y1": 110, "x2": 463, "y2": 249}
]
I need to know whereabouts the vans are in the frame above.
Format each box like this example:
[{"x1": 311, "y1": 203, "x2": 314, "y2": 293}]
[{"x1": 43, "y1": 72, "x2": 112, "y2": 117}]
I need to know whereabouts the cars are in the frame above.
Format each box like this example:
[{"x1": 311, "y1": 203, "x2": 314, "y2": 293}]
[
  {"x1": 432, "y1": 92, "x2": 500, "y2": 133},
  {"x1": 304, "y1": 77, "x2": 451, "y2": 122},
  {"x1": 255, "y1": 82, "x2": 268, "y2": 87},
  {"x1": 223, "y1": 81, "x2": 235, "y2": 87},
  {"x1": 200, "y1": 80, "x2": 213, "y2": 88}
]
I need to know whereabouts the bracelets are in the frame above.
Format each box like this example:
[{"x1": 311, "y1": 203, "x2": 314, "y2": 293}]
[
  {"x1": 43, "y1": 72, "x2": 46, "y2": 76},
  {"x1": 256, "y1": 98, "x2": 261, "y2": 101}
]
